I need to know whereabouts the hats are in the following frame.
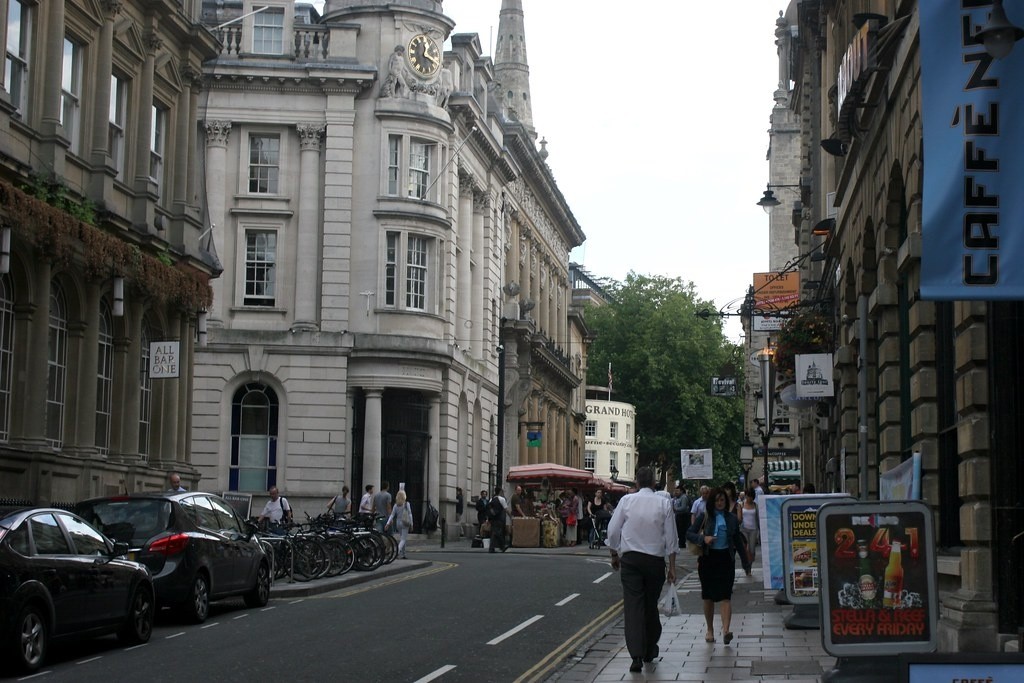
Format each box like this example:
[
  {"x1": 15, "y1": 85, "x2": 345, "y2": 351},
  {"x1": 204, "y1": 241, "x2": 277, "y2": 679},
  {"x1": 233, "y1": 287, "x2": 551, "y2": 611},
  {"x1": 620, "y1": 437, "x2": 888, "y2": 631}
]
[
  {"x1": 571, "y1": 487, "x2": 578, "y2": 495},
  {"x1": 721, "y1": 482, "x2": 735, "y2": 489}
]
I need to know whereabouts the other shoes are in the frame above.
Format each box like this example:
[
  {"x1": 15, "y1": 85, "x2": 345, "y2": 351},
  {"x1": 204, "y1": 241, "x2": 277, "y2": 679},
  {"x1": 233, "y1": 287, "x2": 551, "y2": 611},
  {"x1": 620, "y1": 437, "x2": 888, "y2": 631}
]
[
  {"x1": 502, "y1": 545, "x2": 510, "y2": 552},
  {"x1": 402, "y1": 555, "x2": 408, "y2": 559},
  {"x1": 724, "y1": 632, "x2": 733, "y2": 644},
  {"x1": 571, "y1": 541, "x2": 576, "y2": 546},
  {"x1": 460, "y1": 534, "x2": 464, "y2": 537},
  {"x1": 679, "y1": 544, "x2": 687, "y2": 548},
  {"x1": 630, "y1": 655, "x2": 643, "y2": 672},
  {"x1": 705, "y1": 629, "x2": 714, "y2": 642},
  {"x1": 643, "y1": 645, "x2": 659, "y2": 662},
  {"x1": 489, "y1": 547, "x2": 495, "y2": 553}
]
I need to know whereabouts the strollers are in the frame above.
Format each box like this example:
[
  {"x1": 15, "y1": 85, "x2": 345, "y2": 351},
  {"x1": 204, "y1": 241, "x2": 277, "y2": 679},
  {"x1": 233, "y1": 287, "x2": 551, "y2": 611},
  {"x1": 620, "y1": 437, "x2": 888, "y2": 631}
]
[{"x1": 589, "y1": 509, "x2": 612, "y2": 550}]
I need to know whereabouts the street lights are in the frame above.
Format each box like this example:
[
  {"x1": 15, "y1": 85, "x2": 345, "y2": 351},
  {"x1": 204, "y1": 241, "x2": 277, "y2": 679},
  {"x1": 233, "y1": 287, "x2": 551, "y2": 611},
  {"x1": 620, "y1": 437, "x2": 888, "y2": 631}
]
[{"x1": 739, "y1": 431, "x2": 755, "y2": 491}]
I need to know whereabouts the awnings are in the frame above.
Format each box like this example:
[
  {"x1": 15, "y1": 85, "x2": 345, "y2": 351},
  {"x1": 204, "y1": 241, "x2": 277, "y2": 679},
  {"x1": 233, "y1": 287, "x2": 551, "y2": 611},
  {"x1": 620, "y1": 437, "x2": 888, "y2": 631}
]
[{"x1": 763, "y1": 460, "x2": 802, "y2": 482}]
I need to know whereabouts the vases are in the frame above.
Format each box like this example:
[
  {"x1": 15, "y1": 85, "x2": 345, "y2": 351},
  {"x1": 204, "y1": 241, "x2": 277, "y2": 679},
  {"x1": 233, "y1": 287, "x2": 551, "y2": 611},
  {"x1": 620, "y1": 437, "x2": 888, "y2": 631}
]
[{"x1": 775, "y1": 380, "x2": 815, "y2": 409}]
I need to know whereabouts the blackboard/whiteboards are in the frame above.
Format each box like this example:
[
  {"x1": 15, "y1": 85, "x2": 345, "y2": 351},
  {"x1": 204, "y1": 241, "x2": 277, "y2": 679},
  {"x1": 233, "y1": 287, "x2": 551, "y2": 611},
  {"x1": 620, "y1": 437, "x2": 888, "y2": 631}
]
[{"x1": 222, "y1": 492, "x2": 252, "y2": 520}]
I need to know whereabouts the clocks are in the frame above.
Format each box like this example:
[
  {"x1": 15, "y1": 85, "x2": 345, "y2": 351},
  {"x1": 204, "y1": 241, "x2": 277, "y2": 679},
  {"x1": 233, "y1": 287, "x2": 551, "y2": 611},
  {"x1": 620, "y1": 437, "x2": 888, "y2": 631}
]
[{"x1": 408, "y1": 33, "x2": 440, "y2": 74}]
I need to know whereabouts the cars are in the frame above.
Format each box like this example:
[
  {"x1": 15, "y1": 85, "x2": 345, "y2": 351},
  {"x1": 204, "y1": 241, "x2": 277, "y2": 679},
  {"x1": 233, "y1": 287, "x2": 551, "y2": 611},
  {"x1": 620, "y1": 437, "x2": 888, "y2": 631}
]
[
  {"x1": 0, "y1": 506, "x2": 157, "y2": 674},
  {"x1": 75, "y1": 488, "x2": 271, "y2": 626}
]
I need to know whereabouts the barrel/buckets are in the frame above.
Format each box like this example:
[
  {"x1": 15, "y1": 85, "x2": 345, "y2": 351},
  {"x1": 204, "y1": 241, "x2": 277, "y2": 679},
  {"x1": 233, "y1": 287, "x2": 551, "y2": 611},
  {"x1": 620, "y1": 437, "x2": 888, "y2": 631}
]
[{"x1": 482, "y1": 539, "x2": 490, "y2": 548}]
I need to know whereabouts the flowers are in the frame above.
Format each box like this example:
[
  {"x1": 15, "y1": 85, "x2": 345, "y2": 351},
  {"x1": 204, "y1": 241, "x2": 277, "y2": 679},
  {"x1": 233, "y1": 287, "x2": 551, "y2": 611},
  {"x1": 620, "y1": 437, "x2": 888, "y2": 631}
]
[{"x1": 771, "y1": 312, "x2": 834, "y2": 378}]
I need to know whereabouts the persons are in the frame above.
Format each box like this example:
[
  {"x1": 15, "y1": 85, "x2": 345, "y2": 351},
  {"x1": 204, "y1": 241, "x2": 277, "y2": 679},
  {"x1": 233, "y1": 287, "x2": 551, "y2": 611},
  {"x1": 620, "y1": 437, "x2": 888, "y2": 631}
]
[
  {"x1": 555, "y1": 489, "x2": 618, "y2": 548},
  {"x1": 511, "y1": 485, "x2": 535, "y2": 517},
  {"x1": 389, "y1": 45, "x2": 409, "y2": 98},
  {"x1": 327, "y1": 486, "x2": 351, "y2": 517},
  {"x1": 168, "y1": 474, "x2": 186, "y2": 491},
  {"x1": 723, "y1": 479, "x2": 765, "y2": 576},
  {"x1": 372, "y1": 482, "x2": 393, "y2": 554},
  {"x1": 456, "y1": 487, "x2": 464, "y2": 537},
  {"x1": 792, "y1": 479, "x2": 800, "y2": 493},
  {"x1": 486, "y1": 487, "x2": 511, "y2": 553},
  {"x1": 384, "y1": 490, "x2": 413, "y2": 559},
  {"x1": 439, "y1": 61, "x2": 453, "y2": 108},
  {"x1": 359, "y1": 484, "x2": 374, "y2": 516},
  {"x1": 476, "y1": 490, "x2": 489, "y2": 534},
  {"x1": 604, "y1": 467, "x2": 680, "y2": 672},
  {"x1": 686, "y1": 487, "x2": 751, "y2": 644},
  {"x1": 258, "y1": 486, "x2": 291, "y2": 534},
  {"x1": 655, "y1": 483, "x2": 709, "y2": 548}
]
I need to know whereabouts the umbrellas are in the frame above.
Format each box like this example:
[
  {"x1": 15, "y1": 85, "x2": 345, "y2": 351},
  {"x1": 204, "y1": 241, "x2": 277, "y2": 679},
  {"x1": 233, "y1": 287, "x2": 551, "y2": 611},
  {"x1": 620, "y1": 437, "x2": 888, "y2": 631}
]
[{"x1": 507, "y1": 463, "x2": 636, "y2": 499}]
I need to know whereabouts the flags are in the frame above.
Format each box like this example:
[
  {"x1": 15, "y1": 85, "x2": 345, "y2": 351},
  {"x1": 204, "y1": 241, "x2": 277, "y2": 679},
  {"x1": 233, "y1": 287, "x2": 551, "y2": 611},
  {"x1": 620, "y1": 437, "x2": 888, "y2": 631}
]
[{"x1": 608, "y1": 369, "x2": 612, "y2": 391}]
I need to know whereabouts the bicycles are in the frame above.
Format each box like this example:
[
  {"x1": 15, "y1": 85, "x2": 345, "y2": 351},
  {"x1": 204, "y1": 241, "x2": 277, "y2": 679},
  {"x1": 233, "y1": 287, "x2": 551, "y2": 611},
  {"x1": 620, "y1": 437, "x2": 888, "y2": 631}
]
[{"x1": 246, "y1": 511, "x2": 399, "y2": 582}]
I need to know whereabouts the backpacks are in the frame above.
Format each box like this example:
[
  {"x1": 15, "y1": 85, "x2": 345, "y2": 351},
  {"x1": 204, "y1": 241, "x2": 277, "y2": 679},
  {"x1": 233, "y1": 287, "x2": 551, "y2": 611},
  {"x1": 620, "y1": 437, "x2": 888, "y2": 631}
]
[{"x1": 486, "y1": 498, "x2": 500, "y2": 517}]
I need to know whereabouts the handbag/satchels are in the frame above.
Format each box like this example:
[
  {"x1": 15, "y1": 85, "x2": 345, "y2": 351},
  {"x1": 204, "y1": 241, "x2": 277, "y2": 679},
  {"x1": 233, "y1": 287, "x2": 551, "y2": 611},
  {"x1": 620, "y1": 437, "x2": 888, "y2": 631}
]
[
  {"x1": 657, "y1": 581, "x2": 681, "y2": 616},
  {"x1": 688, "y1": 511, "x2": 708, "y2": 556},
  {"x1": 566, "y1": 512, "x2": 576, "y2": 525},
  {"x1": 480, "y1": 521, "x2": 491, "y2": 538},
  {"x1": 402, "y1": 502, "x2": 409, "y2": 527},
  {"x1": 472, "y1": 539, "x2": 484, "y2": 548},
  {"x1": 280, "y1": 496, "x2": 293, "y2": 522}
]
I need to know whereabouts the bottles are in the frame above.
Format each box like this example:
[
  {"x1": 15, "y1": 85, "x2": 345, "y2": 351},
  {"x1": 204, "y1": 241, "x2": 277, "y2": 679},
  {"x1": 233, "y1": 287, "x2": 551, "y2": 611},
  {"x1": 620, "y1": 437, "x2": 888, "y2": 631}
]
[
  {"x1": 883, "y1": 541, "x2": 904, "y2": 608},
  {"x1": 856, "y1": 539, "x2": 881, "y2": 609}
]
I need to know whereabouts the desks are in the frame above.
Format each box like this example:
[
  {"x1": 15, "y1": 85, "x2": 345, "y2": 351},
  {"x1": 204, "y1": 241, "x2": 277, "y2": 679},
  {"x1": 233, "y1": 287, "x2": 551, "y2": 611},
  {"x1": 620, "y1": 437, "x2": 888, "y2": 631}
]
[{"x1": 511, "y1": 519, "x2": 563, "y2": 546}]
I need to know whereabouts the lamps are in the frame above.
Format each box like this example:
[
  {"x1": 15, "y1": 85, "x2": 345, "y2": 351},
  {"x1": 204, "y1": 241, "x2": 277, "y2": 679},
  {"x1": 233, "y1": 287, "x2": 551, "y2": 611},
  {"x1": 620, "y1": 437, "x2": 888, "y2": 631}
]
[
  {"x1": 804, "y1": 280, "x2": 821, "y2": 289},
  {"x1": 756, "y1": 184, "x2": 800, "y2": 214},
  {"x1": 818, "y1": 132, "x2": 842, "y2": 157},
  {"x1": 496, "y1": 345, "x2": 504, "y2": 353},
  {"x1": 113, "y1": 273, "x2": 124, "y2": 316},
  {"x1": 292, "y1": 328, "x2": 297, "y2": 333},
  {"x1": 0, "y1": 223, "x2": 12, "y2": 274},
  {"x1": 811, "y1": 217, "x2": 837, "y2": 235},
  {"x1": 195, "y1": 310, "x2": 208, "y2": 348},
  {"x1": 340, "y1": 329, "x2": 348, "y2": 333},
  {"x1": 973, "y1": 0, "x2": 1024, "y2": 60},
  {"x1": 810, "y1": 251, "x2": 825, "y2": 262}
]
